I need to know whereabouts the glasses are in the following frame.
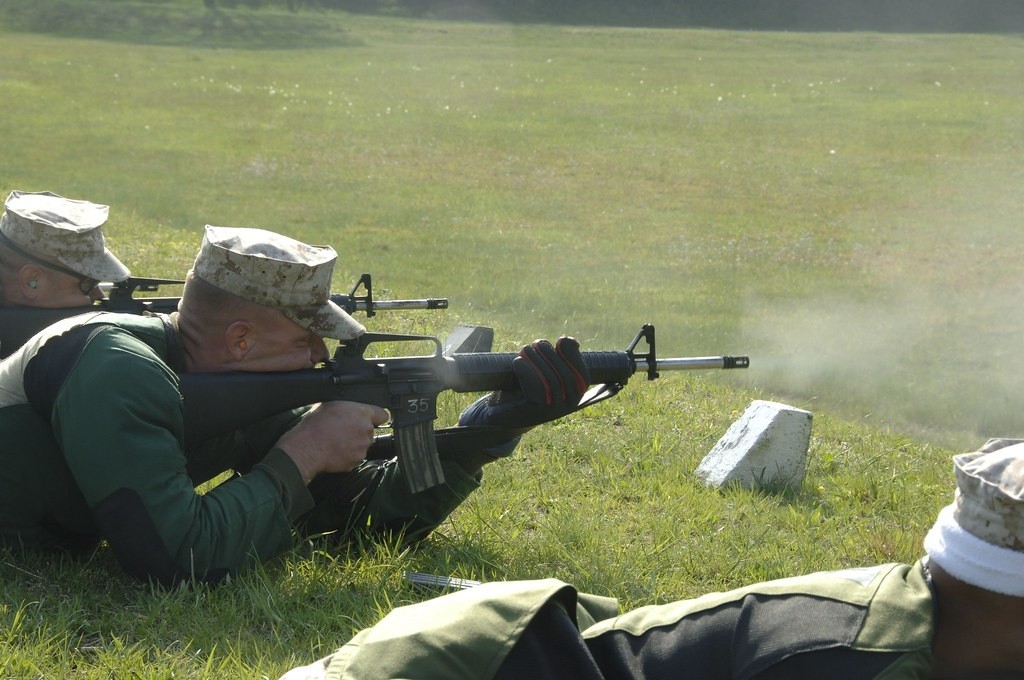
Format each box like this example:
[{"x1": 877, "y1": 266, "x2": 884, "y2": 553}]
[{"x1": 78, "y1": 275, "x2": 101, "y2": 294}]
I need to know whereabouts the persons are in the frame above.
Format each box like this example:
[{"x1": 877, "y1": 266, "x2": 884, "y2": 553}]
[
  {"x1": 273, "y1": 433, "x2": 1024, "y2": 680},
  {"x1": 0, "y1": 224, "x2": 591, "y2": 590},
  {"x1": 1, "y1": 187, "x2": 136, "y2": 364}
]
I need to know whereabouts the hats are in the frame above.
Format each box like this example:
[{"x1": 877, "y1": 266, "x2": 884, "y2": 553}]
[
  {"x1": 194, "y1": 224, "x2": 366, "y2": 340},
  {"x1": 952, "y1": 437, "x2": 1024, "y2": 554},
  {"x1": 0, "y1": 189, "x2": 132, "y2": 283}
]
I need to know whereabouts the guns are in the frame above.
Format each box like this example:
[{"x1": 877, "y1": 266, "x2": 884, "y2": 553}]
[
  {"x1": 185, "y1": 321, "x2": 753, "y2": 498},
  {"x1": 1, "y1": 275, "x2": 448, "y2": 377}
]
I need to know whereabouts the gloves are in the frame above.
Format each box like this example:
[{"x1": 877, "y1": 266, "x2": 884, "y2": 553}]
[{"x1": 459, "y1": 336, "x2": 588, "y2": 459}]
